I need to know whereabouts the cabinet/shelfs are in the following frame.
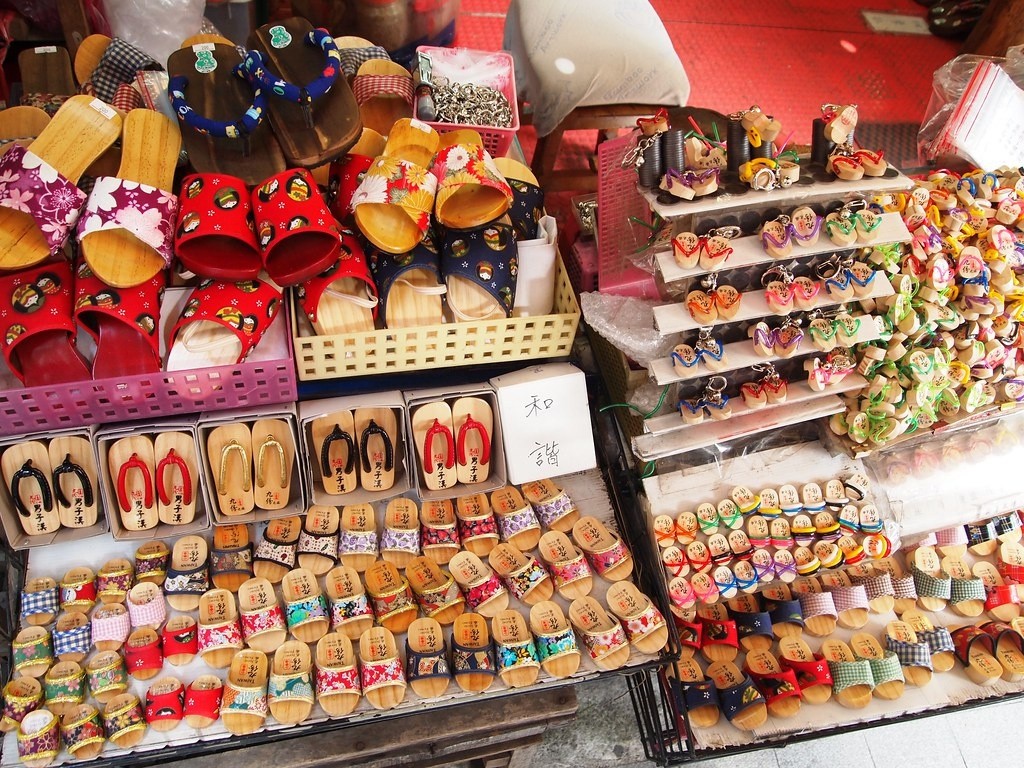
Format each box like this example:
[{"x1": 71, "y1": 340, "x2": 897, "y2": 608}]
[{"x1": 598, "y1": 157, "x2": 917, "y2": 469}]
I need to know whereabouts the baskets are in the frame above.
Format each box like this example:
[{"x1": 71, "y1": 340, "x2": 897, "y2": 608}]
[
  {"x1": 291, "y1": 244, "x2": 581, "y2": 380},
  {"x1": 1, "y1": 297, "x2": 296, "y2": 439},
  {"x1": 567, "y1": 238, "x2": 598, "y2": 308},
  {"x1": 586, "y1": 321, "x2": 654, "y2": 471},
  {"x1": 595, "y1": 129, "x2": 660, "y2": 303}
]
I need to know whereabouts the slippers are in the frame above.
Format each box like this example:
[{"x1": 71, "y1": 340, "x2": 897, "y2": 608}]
[
  {"x1": 411, "y1": 401, "x2": 458, "y2": 489},
  {"x1": 205, "y1": 422, "x2": 256, "y2": 516},
  {"x1": 153, "y1": 430, "x2": 198, "y2": 526},
  {"x1": 47, "y1": 434, "x2": 100, "y2": 529},
  {"x1": 652, "y1": 105, "x2": 1024, "y2": 737},
  {"x1": 107, "y1": 435, "x2": 160, "y2": 531},
  {"x1": 0, "y1": 441, "x2": 60, "y2": 535},
  {"x1": 1, "y1": 475, "x2": 668, "y2": 757},
  {"x1": 354, "y1": 408, "x2": 396, "y2": 493},
  {"x1": 312, "y1": 411, "x2": 358, "y2": 495},
  {"x1": 0, "y1": 16, "x2": 545, "y2": 389},
  {"x1": 451, "y1": 396, "x2": 494, "y2": 485},
  {"x1": 252, "y1": 419, "x2": 294, "y2": 511}
]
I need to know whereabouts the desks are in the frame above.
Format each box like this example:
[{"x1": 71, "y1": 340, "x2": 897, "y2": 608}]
[{"x1": 154, "y1": 683, "x2": 579, "y2": 768}]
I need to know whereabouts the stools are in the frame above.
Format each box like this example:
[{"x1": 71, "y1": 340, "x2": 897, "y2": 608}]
[{"x1": 503, "y1": 0, "x2": 691, "y2": 193}]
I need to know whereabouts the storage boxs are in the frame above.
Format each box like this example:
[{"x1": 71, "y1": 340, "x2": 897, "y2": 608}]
[
  {"x1": 298, "y1": 389, "x2": 413, "y2": 508},
  {"x1": 0, "y1": 423, "x2": 112, "y2": 552},
  {"x1": 91, "y1": 414, "x2": 213, "y2": 542},
  {"x1": 196, "y1": 400, "x2": 309, "y2": 528},
  {"x1": 494, "y1": 361, "x2": 599, "y2": 487},
  {"x1": 404, "y1": 382, "x2": 508, "y2": 502}
]
